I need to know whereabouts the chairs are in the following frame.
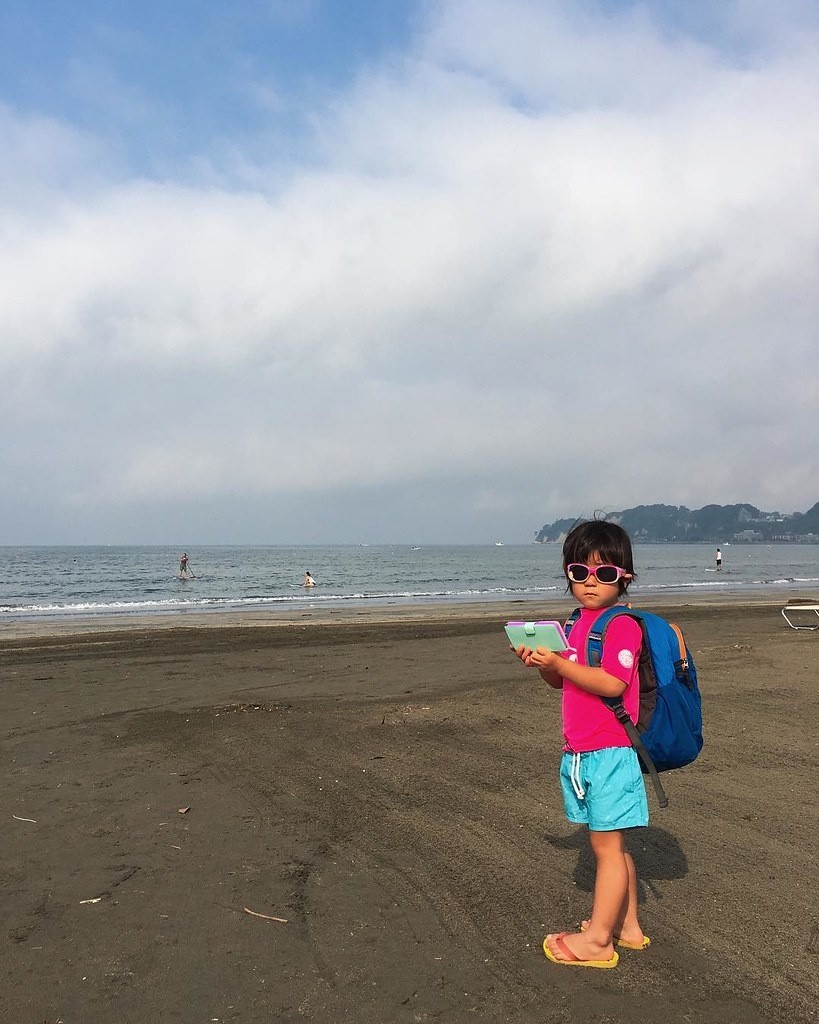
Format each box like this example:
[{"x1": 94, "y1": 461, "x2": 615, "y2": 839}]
[{"x1": 781, "y1": 599, "x2": 819, "y2": 630}]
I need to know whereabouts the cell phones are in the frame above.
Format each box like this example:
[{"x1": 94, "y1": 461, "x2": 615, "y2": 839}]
[{"x1": 504, "y1": 621, "x2": 570, "y2": 651}]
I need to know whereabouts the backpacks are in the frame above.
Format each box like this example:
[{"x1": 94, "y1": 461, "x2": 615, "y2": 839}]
[{"x1": 565, "y1": 603, "x2": 704, "y2": 774}]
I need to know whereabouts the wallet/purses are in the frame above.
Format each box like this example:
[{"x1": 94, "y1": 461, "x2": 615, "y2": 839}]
[{"x1": 505, "y1": 621, "x2": 571, "y2": 652}]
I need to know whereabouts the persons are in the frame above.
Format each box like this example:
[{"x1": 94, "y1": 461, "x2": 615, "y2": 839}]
[
  {"x1": 180, "y1": 553, "x2": 189, "y2": 578},
  {"x1": 713, "y1": 549, "x2": 721, "y2": 570},
  {"x1": 303, "y1": 571, "x2": 316, "y2": 587},
  {"x1": 510, "y1": 520, "x2": 649, "y2": 968}
]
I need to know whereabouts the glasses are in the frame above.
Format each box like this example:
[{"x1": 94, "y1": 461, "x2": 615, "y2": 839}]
[{"x1": 566, "y1": 563, "x2": 632, "y2": 584}]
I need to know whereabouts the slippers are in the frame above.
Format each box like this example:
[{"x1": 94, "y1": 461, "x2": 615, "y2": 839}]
[
  {"x1": 580, "y1": 926, "x2": 651, "y2": 949},
  {"x1": 543, "y1": 933, "x2": 619, "y2": 968}
]
